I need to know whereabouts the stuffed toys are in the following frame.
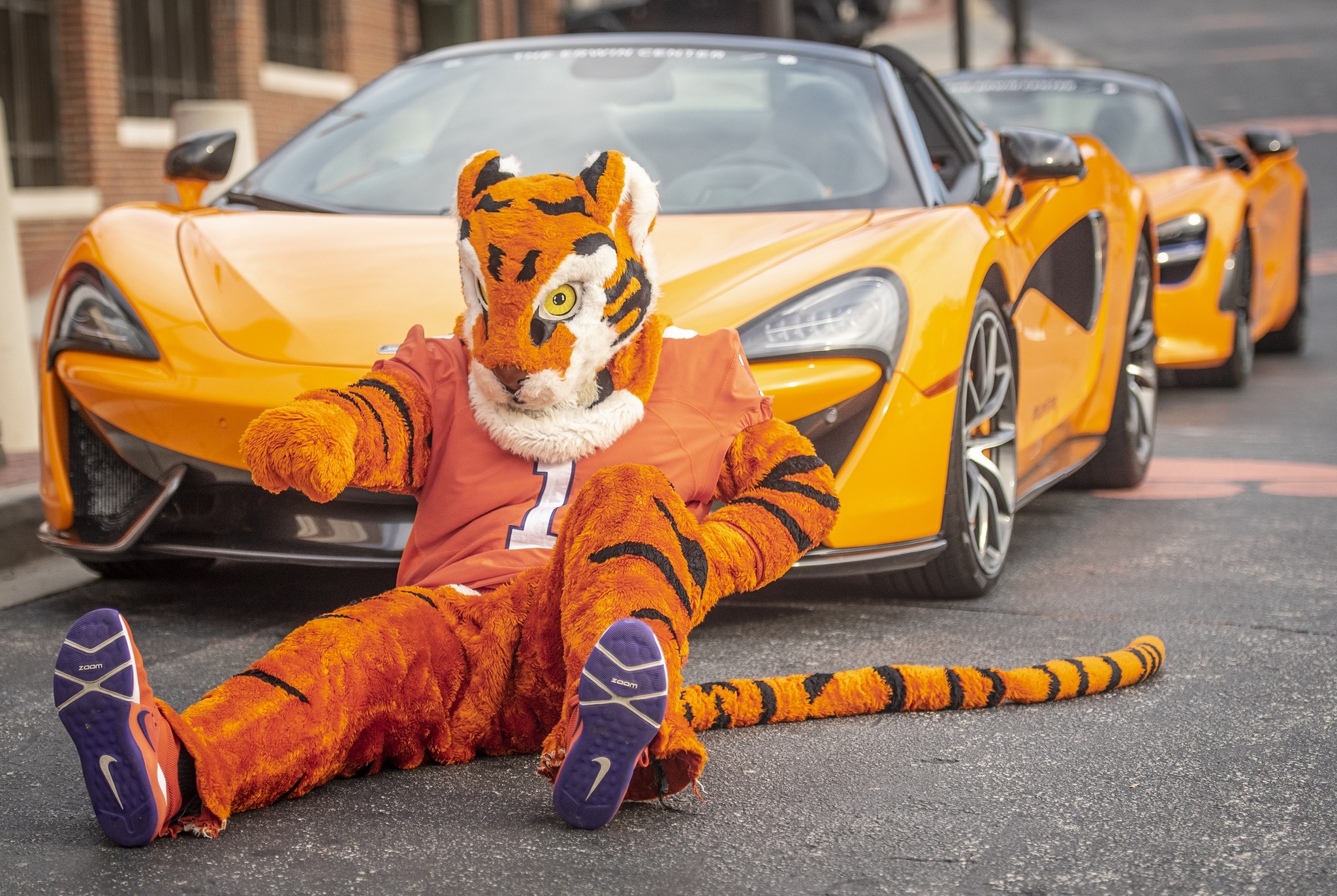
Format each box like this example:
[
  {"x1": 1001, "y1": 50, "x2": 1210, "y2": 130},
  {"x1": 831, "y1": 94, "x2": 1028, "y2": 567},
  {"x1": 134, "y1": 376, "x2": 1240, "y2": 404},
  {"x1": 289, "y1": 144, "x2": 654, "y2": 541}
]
[{"x1": 52, "y1": 149, "x2": 1163, "y2": 851}]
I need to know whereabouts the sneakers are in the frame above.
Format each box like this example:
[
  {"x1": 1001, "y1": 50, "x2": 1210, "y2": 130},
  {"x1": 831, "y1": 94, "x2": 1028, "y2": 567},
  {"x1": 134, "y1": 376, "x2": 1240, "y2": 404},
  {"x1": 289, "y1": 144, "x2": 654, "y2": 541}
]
[
  {"x1": 552, "y1": 618, "x2": 668, "y2": 830},
  {"x1": 53, "y1": 609, "x2": 182, "y2": 848}
]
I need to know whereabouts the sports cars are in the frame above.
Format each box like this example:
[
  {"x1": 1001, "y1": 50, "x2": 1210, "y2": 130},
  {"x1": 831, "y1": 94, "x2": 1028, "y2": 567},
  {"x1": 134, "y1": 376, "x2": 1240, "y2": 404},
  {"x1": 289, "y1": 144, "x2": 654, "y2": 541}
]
[
  {"x1": 924, "y1": 63, "x2": 1314, "y2": 390},
  {"x1": 36, "y1": 30, "x2": 1164, "y2": 610}
]
